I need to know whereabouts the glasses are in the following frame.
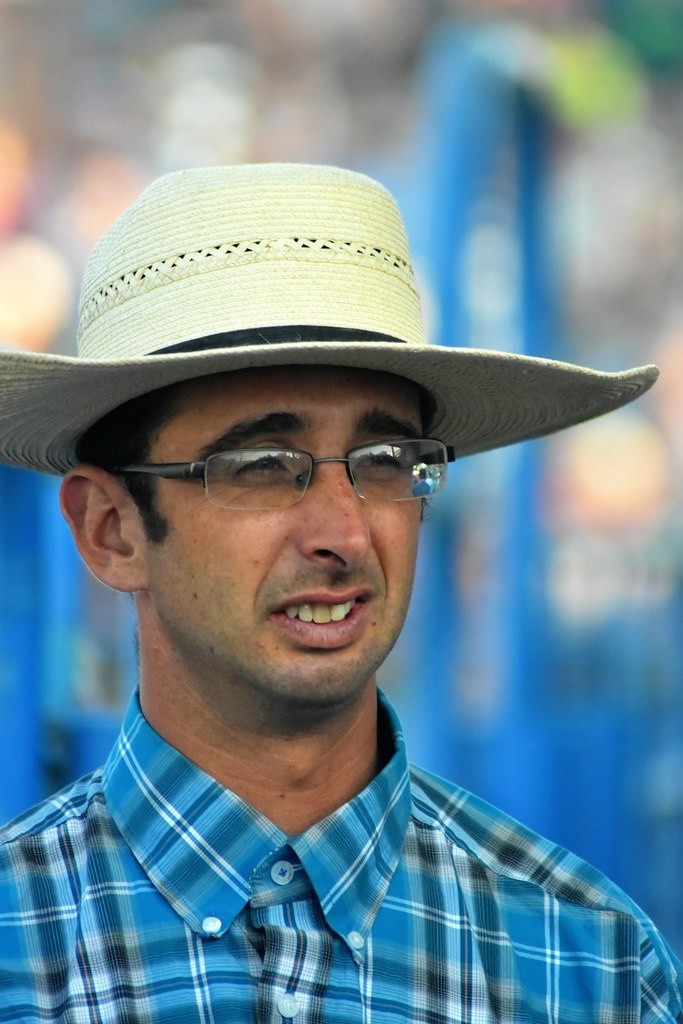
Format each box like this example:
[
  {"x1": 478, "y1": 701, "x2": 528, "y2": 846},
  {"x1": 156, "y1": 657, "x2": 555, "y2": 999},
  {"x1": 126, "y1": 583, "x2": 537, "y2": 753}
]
[{"x1": 104, "y1": 436, "x2": 454, "y2": 511}]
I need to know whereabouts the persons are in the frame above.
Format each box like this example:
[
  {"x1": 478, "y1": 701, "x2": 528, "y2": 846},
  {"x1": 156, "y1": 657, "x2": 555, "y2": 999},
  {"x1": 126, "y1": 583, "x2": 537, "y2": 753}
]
[
  {"x1": 0, "y1": 158, "x2": 683, "y2": 1024},
  {"x1": 412, "y1": 468, "x2": 430, "y2": 496}
]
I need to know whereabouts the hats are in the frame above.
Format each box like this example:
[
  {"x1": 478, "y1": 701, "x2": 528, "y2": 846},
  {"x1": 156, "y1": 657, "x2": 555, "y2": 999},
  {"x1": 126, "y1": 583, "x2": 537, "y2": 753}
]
[{"x1": 0, "y1": 162, "x2": 660, "y2": 472}]
